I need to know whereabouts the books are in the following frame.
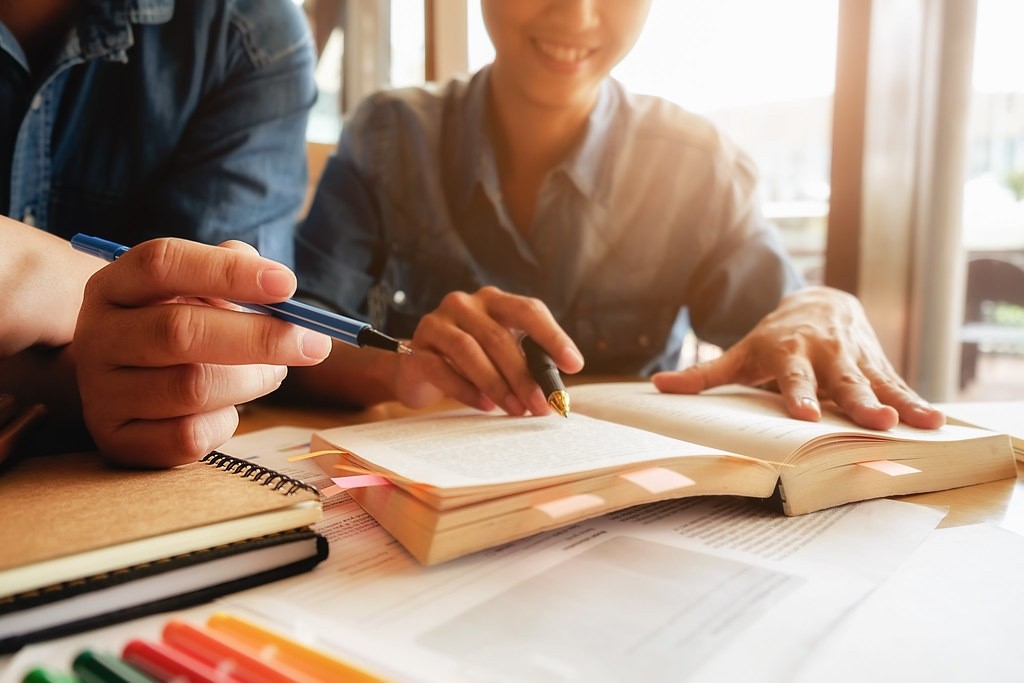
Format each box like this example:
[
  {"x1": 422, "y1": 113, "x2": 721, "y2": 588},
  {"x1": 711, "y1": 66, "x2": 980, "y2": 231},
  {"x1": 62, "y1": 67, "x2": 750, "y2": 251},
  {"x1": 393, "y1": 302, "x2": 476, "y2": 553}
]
[
  {"x1": 0, "y1": 527, "x2": 331, "y2": 658},
  {"x1": 0, "y1": 450, "x2": 325, "y2": 599},
  {"x1": 311, "y1": 382, "x2": 1019, "y2": 566},
  {"x1": 930, "y1": 403, "x2": 1024, "y2": 466}
]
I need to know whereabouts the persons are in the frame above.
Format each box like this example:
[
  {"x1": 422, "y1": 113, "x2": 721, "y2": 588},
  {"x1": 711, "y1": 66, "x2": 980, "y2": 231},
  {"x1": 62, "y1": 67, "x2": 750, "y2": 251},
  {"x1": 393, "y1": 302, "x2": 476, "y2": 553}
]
[
  {"x1": 1, "y1": 0, "x2": 331, "y2": 470},
  {"x1": 274, "y1": 1, "x2": 946, "y2": 431}
]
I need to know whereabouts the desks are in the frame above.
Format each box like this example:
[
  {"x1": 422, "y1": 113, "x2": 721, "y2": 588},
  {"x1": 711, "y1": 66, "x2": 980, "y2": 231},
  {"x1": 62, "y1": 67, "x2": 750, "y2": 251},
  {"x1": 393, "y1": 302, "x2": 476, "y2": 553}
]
[{"x1": 0, "y1": 376, "x2": 1024, "y2": 683}]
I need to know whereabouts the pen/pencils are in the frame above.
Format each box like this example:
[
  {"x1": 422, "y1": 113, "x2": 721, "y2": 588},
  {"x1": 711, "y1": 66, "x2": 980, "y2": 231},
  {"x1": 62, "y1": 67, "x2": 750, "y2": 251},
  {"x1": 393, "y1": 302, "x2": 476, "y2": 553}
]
[
  {"x1": 71, "y1": 650, "x2": 160, "y2": 683},
  {"x1": 120, "y1": 638, "x2": 231, "y2": 683},
  {"x1": 513, "y1": 328, "x2": 571, "y2": 422},
  {"x1": 162, "y1": 620, "x2": 300, "y2": 683},
  {"x1": 69, "y1": 233, "x2": 416, "y2": 356},
  {"x1": 210, "y1": 613, "x2": 380, "y2": 682},
  {"x1": 22, "y1": 668, "x2": 71, "y2": 683}
]
